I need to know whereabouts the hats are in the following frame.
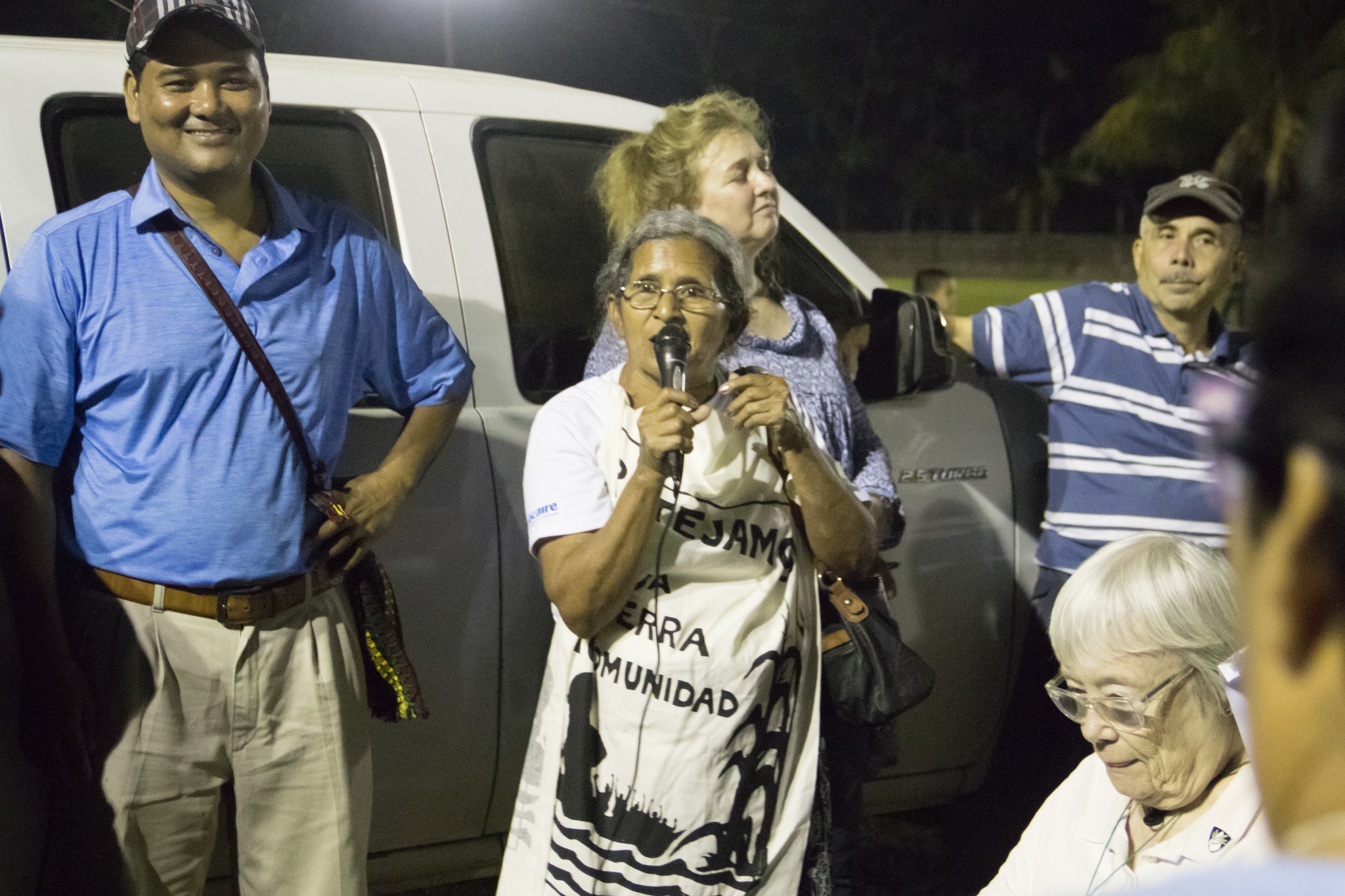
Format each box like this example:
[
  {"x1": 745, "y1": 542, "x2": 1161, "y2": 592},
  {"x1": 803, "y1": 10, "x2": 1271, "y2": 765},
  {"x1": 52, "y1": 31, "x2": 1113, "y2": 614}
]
[
  {"x1": 1144, "y1": 171, "x2": 1245, "y2": 221},
  {"x1": 125, "y1": 0, "x2": 266, "y2": 63}
]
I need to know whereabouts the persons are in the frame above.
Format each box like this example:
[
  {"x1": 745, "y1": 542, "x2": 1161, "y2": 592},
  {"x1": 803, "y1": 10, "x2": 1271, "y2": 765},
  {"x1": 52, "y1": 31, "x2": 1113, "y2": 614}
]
[
  {"x1": 914, "y1": 269, "x2": 957, "y2": 317},
  {"x1": 582, "y1": 92, "x2": 906, "y2": 896},
  {"x1": 494, "y1": 210, "x2": 881, "y2": 896},
  {"x1": 978, "y1": 530, "x2": 1269, "y2": 896},
  {"x1": 1106, "y1": 147, "x2": 1345, "y2": 895},
  {"x1": 1, "y1": 0, "x2": 477, "y2": 896},
  {"x1": 840, "y1": 171, "x2": 1259, "y2": 746}
]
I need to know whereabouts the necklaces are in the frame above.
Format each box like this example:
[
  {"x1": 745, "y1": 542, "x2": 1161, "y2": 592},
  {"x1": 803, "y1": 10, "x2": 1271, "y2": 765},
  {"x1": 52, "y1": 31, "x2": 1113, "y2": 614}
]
[{"x1": 1085, "y1": 800, "x2": 1180, "y2": 896}]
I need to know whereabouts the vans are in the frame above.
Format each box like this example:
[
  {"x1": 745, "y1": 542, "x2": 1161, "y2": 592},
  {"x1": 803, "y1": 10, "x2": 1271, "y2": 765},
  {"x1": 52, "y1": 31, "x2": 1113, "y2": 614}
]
[{"x1": 0, "y1": 34, "x2": 1050, "y2": 896}]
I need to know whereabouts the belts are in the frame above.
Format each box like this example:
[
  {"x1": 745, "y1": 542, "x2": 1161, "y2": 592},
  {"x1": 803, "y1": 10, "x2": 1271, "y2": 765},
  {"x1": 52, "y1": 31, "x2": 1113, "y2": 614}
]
[{"x1": 99, "y1": 562, "x2": 332, "y2": 629}]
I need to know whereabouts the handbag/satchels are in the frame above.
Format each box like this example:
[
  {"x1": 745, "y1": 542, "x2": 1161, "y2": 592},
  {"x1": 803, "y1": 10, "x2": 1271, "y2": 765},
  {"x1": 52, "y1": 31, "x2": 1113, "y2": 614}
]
[
  {"x1": 763, "y1": 418, "x2": 936, "y2": 725},
  {"x1": 314, "y1": 499, "x2": 430, "y2": 721}
]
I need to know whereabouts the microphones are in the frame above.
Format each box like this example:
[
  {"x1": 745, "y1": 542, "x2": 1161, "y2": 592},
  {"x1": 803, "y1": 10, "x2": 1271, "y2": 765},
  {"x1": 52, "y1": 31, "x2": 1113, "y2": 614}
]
[{"x1": 655, "y1": 323, "x2": 691, "y2": 482}]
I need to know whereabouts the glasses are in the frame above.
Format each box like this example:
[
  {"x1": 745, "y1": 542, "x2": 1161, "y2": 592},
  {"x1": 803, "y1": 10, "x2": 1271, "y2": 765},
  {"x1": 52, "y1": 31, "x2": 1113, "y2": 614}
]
[
  {"x1": 1046, "y1": 663, "x2": 1197, "y2": 736},
  {"x1": 618, "y1": 282, "x2": 735, "y2": 311}
]
